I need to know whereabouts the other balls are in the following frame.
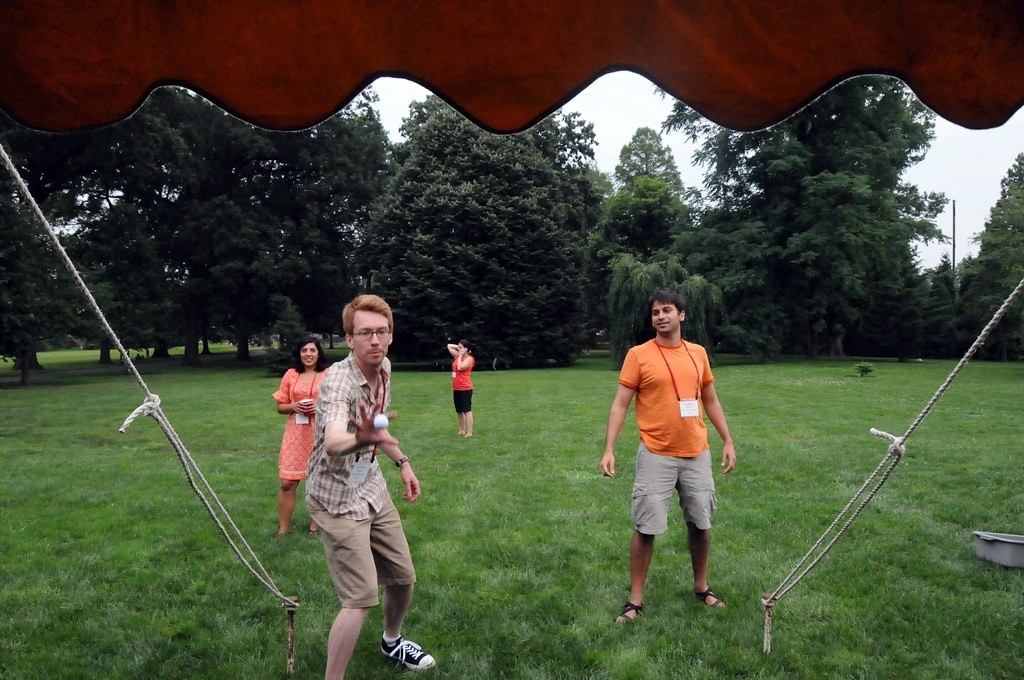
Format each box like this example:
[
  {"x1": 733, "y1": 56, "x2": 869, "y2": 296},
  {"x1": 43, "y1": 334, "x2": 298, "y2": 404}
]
[{"x1": 374, "y1": 414, "x2": 389, "y2": 429}]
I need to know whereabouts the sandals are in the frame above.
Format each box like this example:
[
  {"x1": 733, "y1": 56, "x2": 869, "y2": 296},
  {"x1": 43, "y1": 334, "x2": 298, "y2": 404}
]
[
  {"x1": 694, "y1": 585, "x2": 726, "y2": 608},
  {"x1": 614, "y1": 602, "x2": 644, "y2": 623}
]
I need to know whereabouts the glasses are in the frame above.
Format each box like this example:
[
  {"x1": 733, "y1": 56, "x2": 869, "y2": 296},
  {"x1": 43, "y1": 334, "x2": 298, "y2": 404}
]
[{"x1": 351, "y1": 328, "x2": 391, "y2": 340}]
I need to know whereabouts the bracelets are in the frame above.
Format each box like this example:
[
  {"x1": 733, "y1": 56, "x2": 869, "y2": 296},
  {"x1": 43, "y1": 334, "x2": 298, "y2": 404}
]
[{"x1": 396, "y1": 456, "x2": 410, "y2": 467}]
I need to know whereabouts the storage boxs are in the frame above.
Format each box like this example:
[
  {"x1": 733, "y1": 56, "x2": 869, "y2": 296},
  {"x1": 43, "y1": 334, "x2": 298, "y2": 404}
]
[{"x1": 973, "y1": 530, "x2": 1024, "y2": 568}]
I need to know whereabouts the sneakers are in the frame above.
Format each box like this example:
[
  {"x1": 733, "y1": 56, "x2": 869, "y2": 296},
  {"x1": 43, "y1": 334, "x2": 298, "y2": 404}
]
[{"x1": 381, "y1": 631, "x2": 436, "y2": 672}]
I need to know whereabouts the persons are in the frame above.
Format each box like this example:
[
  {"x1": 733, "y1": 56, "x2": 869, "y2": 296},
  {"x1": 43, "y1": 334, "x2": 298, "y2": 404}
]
[
  {"x1": 448, "y1": 340, "x2": 475, "y2": 437},
  {"x1": 272, "y1": 338, "x2": 331, "y2": 534},
  {"x1": 600, "y1": 291, "x2": 735, "y2": 623},
  {"x1": 304, "y1": 295, "x2": 435, "y2": 680}
]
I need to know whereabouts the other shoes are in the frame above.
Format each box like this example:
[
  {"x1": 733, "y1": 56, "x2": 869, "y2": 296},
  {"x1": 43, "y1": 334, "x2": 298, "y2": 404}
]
[
  {"x1": 274, "y1": 527, "x2": 292, "y2": 539},
  {"x1": 308, "y1": 524, "x2": 319, "y2": 536}
]
